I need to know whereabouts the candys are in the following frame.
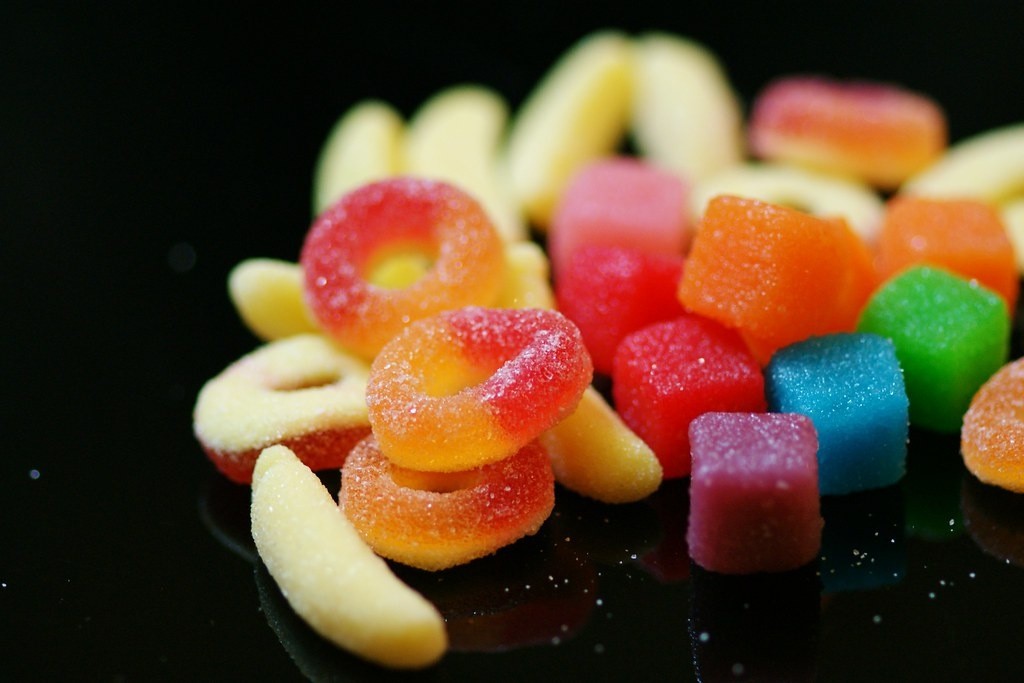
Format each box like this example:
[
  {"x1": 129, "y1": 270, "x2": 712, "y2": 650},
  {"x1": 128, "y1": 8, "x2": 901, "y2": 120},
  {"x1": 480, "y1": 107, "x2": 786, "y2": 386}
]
[{"x1": 187, "y1": 33, "x2": 1024, "y2": 663}]
